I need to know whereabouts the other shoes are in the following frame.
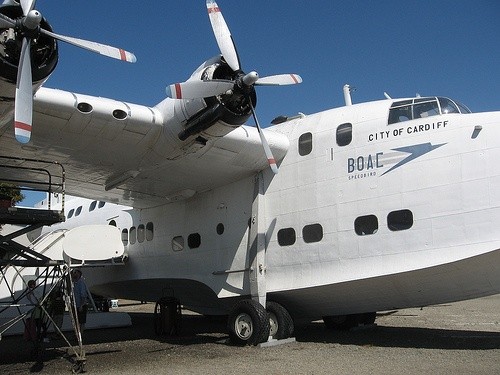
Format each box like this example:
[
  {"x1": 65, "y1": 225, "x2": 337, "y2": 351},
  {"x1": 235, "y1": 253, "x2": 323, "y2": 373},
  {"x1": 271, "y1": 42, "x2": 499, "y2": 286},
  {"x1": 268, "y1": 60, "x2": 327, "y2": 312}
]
[{"x1": 44, "y1": 338, "x2": 50, "y2": 343}]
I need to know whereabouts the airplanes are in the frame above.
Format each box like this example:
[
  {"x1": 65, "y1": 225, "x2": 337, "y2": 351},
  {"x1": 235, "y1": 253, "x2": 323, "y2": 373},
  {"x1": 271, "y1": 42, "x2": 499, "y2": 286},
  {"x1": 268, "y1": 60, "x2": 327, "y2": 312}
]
[{"x1": 0, "y1": 0, "x2": 500, "y2": 347}]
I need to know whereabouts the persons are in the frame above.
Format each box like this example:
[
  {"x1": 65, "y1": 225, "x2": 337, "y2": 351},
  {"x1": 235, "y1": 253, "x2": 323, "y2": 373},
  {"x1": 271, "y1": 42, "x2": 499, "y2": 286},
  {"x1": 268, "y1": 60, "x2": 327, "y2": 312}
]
[
  {"x1": 71, "y1": 268, "x2": 87, "y2": 344},
  {"x1": 23, "y1": 280, "x2": 65, "y2": 345}
]
